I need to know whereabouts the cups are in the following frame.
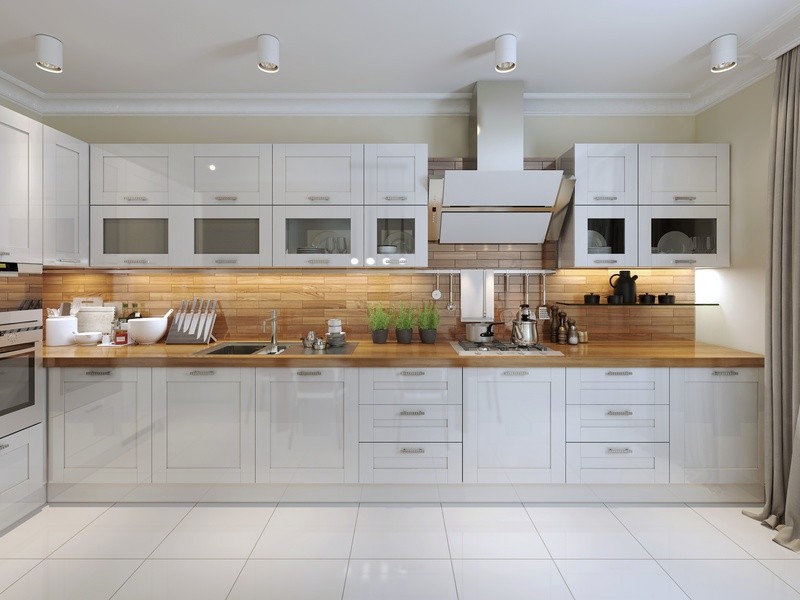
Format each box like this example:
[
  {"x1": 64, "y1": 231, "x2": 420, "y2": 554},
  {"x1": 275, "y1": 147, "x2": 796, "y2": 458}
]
[
  {"x1": 584, "y1": 292, "x2": 600, "y2": 304},
  {"x1": 311, "y1": 231, "x2": 347, "y2": 254},
  {"x1": 327, "y1": 319, "x2": 342, "y2": 333},
  {"x1": 690, "y1": 236, "x2": 714, "y2": 254},
  {"x1": 658, "y1": 293, "x2": 675, "y2": 304},
  {"x1": 607, "y1": 295, "x2": 623, "y2": 304},
  {"x1": 577, "y1": 331, "x2": 588, "y2": 342},
  {"x1": 639, "y1": 293, "x2": 656, "y2": 304}
]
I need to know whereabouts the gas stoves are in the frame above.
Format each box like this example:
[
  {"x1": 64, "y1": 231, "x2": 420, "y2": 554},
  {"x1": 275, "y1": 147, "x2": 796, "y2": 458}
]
[{"x1": 449, "y1": 339, "x2": 565, "y2": 357}]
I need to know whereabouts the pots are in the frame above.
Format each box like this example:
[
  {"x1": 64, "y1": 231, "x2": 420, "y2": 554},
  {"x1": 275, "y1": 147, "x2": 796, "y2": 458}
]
[
  {"x1": 466, "y1": 322, "x2": 505, "y2": 343},
  {"x1": 46, "y1": 316, "x2": 77, "y2": 347},
  {"x1": 511, "y1": 314, "x2": 538, "y2": 347},
  {"x1": 77, "y1": 306, "x2": 114, "y2": 342}
]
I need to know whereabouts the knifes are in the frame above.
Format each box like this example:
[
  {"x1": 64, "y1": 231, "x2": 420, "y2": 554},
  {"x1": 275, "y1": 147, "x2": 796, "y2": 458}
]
[{"x1": 175, "y1": 297, "x2": 218, "y2": 342}]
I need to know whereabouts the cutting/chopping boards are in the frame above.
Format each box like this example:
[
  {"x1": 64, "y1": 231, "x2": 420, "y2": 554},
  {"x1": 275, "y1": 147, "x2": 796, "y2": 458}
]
[{"x1": 71, "y1": 297, "x2": 102, "y2": 314}]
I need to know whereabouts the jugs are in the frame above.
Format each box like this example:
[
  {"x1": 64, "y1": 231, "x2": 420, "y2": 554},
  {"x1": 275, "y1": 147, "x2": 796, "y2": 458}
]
[{"x1": 609, "y1": 271, "x2": 638, "y2": 304}]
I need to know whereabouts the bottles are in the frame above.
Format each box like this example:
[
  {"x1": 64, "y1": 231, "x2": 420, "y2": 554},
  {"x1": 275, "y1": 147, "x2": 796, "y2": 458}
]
[
  {"x1": 550, "y1": 305, "x2": 578, "y2": 345},
  {"x1": 117, "y1": 303, "x2": 131, "y2": 332},
  {"x1": 126, "y1": 303, "x2": 142, "y2": 323}
]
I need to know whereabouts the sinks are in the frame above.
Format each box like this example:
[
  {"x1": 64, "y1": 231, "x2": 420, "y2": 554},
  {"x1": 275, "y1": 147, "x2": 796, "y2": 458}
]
[{"x1": 193, "y1": 341, "x2": 268, "y2": 355}]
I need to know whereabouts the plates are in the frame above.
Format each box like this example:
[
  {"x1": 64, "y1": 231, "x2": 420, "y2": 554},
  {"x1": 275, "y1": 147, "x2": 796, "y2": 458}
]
[
  {"x1": 588, "y1": 230, "x2": 607, "y2": 246},
  {"x1": 296, "y1": 247, "x2": 325, "y2": 254},
  {"x1": 657, "y1": 231, "x2": 693, "y2": 254},
  {"x1": 384, "y1": 231, "x2": 415, "y2": 253}
]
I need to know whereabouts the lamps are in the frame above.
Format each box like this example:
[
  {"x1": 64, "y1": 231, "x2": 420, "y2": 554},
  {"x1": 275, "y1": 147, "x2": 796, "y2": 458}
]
[
  {"x1": 36, "y1": 34, "x2": 64, "y2": 74},
  {"x1": 708, "y1": 33, "x2": 738, "y2": 73},
  {"x1": 493, "y1": 33, "x2": 516, "y2": 73},
  {"x1": 255, "y1": 33, "x2": 279, "y2": 73}
]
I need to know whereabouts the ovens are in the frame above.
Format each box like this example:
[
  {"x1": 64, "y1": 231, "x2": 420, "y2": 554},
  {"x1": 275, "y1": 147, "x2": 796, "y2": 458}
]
[{"x1": 0, "y1": 308, "x2": 43, "y2": 439}]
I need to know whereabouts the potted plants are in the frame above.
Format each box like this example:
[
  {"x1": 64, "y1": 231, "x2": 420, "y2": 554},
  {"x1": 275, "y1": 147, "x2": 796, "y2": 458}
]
[
  {"x1": 392, "y1": 303, "x2": 414, "y2": 344},
  {"x1": 416, "y1": 300, "x2": 441, "y2": 344},
  {"x1": 366, "y1": 303, "x2": 392, "y2": 343}
]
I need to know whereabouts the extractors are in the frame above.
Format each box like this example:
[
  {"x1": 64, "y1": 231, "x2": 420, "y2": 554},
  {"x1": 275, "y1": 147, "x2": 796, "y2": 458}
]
[{"x1": 428, "y1": 78, "x2": 576, "y2": 244}]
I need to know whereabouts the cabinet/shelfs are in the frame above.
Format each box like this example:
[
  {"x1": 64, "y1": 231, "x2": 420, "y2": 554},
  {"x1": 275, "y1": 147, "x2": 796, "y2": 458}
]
[
  {"x1": 669, "y1": 368, "x2": 767, "y2": 503},
  {"x1": 90, "y1": 143, "x2": 427, "y2": 268},
  {"x1": 360, "y1": 366, "x2": 464, "y2": 503},
  {"x1": 462, "y1": 366, "x2": 566, "y2": 503},
  {"x1": 46, "y1": 367, "x2": 152, "y2": 502},
  {"x1": 151, "y1": 367, "x2": 358, "y2": 502},
  {"x1": 566, "y1": 366, "x2": 671, "y2": 502},
  {"x1": 0, "y1": 106, "x2": 89, "y2": 271},
  {"x1": 0, "y1": 422, "x2": 46, "y2": 534},
  {"x1": 49, "y1": 391, "x2": 126, "y2": 483},
  {"x1": 556, "y1": 144, "x2": 731, "y2": 268}
]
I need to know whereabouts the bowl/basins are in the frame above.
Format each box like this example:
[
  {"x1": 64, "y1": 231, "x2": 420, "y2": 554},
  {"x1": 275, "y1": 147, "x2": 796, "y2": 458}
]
[
  {"x1": 73, "y1": 332, "x2": 103, "y2": 346},
  {"x1": 127, "y1": 318, "x2": 168, "y2": 345},
  {"x1": 326, "y1": 333, "x2": 344, "y2": 347},
  {"x1": 378, "y1": 245, "x2": 398, "y2": 253},
  {"x1": 588, "y1": 246, "x2": 612, "y2": 254}
]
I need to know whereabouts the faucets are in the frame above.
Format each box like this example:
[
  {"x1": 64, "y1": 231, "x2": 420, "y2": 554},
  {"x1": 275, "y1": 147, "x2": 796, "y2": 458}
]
[{"x1": 261, "y1": 309, "x2": 279, "y2": 345}]
